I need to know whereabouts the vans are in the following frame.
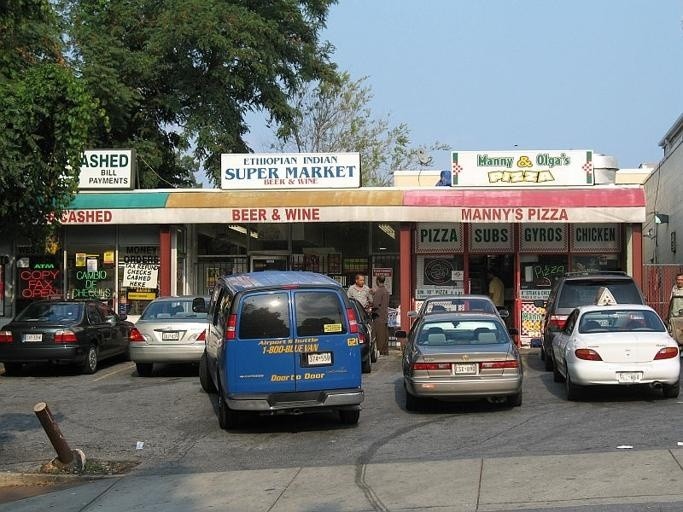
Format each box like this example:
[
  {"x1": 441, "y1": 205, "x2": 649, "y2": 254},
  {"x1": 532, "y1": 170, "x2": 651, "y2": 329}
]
[{"x1": 190, "y1": 269, "x2": 361, "y2": 430}]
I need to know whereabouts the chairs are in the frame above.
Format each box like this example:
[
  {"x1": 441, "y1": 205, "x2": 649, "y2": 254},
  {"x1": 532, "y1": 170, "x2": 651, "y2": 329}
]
[{"x1": 386, "y1": 327, "x2": 406, "y2": 351}]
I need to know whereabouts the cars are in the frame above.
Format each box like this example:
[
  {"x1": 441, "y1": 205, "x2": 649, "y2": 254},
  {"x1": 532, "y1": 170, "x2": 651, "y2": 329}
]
[
  {"x1": 533, "y1": 270, "x2": 681, "y2": 403},
  {"x1": 349, "y1": 298, "x2": 379, "y2": 373},
  {"x1": 394, "y1": 293, "x2": 524, "y2": 408},
  {"x1": 0, "y1": 296, "x2": 211, "y2": 375}
]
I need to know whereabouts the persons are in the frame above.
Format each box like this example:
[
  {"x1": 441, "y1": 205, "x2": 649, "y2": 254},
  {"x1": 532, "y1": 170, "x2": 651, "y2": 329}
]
[
  {"x1": 487, "y1": 269, "x2": 505, "y2": 325},
  {"x1": 345, "y1": 273, "x2": 373, "y2": 321},
  {"x1": 668, "y1": 274, "x2": 683, "y2": 317},
  {"x1": 365, "y1": 275, "x2": 389, "y2": 356}
]
